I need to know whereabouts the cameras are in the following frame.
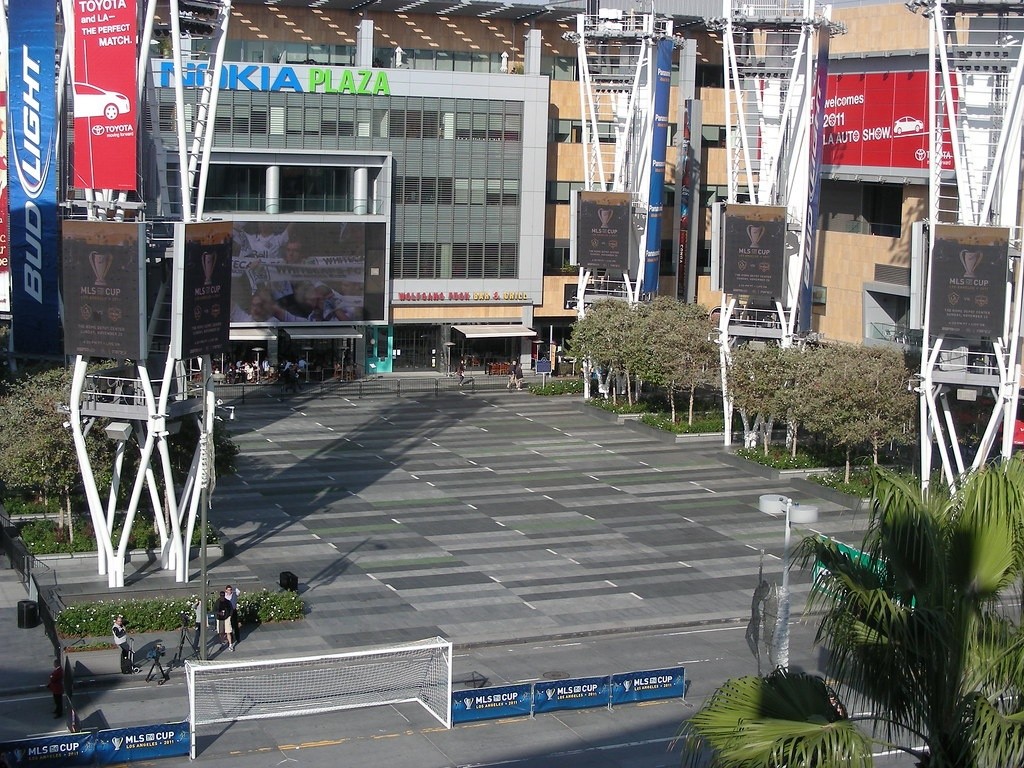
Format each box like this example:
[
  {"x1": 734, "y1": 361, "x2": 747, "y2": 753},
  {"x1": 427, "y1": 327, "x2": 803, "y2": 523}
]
[
  {"x1": 180, "y1": 613, "x2": 190, "y2": 628},
  {"x1": 152, "y1": 644, "x2": 165, "y2": 657}
]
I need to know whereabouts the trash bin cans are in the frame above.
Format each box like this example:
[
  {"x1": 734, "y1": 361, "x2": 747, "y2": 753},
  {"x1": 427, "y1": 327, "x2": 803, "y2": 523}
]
[{"x1": 17, "y1": 600, "x2": 37, "y2": 629}]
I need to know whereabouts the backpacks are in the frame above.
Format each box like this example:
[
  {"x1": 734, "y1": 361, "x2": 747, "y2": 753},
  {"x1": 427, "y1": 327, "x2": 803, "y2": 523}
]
[{"x1": 121, "y1": 659, "x2": 133, "y2": 674}]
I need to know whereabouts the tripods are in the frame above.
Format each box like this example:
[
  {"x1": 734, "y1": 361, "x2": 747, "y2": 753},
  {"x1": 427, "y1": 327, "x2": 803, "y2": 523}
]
[
  {"x1": 172, "y1": 626, "x2": 199, "y2": 664},
  {"x1": 145, "y1": 658, "x2": 168, "y2": 684}
]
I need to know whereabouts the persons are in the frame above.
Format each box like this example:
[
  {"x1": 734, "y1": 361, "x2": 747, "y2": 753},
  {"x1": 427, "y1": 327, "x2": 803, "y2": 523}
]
[
  {"x1": 230, "y1": 220, "x2": 367, "y2": 326},
  {"x1": 514, "y1": 363, "x2": 523, "y2": 391},
  {"x1": 457, "y1": 360, "x2": 466, "y2": 387},
  {"x1": 506, "y1": 360, "x2": 517, "y2": 389},
  {"x1": 46, "y1": 659, "x2": 65, "y2": 719},
  {"x1": 191, "y1": 599, "x2": 201, "y2": 656},
  {"x1": 223, "y1": 584, "x2": 242, "y2": 643},
  {"x1": 211, "y1": 591, "x2": 235, "y2": 652},
  {"x1": 194, "y1": 351, "x2": 359, "y2": 395},
  {"x1": 112, "y1": 616, "x2": 130, "y2": 661}
]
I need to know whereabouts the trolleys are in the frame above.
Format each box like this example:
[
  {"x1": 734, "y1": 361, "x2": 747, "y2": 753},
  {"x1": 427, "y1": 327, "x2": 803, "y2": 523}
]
[{"x1": 127, "y1": 637, "x2": 139, "y2": 674}]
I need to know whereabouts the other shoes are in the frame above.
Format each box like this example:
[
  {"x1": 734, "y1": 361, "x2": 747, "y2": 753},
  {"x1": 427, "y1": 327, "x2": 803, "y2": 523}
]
[
  {"x1": 51, "y1": 709, "x2": 63, "y2": 721},
  {"x1": 237, "y1": 639, "x2": 243, "y2": 644},
  {"x1": 228, "y1": 643, "x2": 233, "y2": 652}
]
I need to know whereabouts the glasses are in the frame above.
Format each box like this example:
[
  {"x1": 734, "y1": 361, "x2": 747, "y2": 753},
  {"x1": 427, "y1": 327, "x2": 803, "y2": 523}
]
[{"x1": 219, "y1": 594, "x2": 226, "y2": 597}]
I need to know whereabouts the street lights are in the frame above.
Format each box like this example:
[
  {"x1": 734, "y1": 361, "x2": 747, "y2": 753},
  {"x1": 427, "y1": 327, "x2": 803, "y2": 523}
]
[
  {"x1": 252, "y1": 347, "x2": 264, "y2": 383},
  {"x1": 337, "y1": 346, "x2": 349, "y2": 382},
  {"x1": 302, "y1": 346, "x2": 314, "y2": 383},
  {"x1": 533, "y1": 340, "x2": 545, "y2": 361},
  {"x1": 759, "y1": 491, "x2": 820, "y2": 677},
  {"x1": 444, "y1": 341, "x2": 457, "y2": 378}
]
